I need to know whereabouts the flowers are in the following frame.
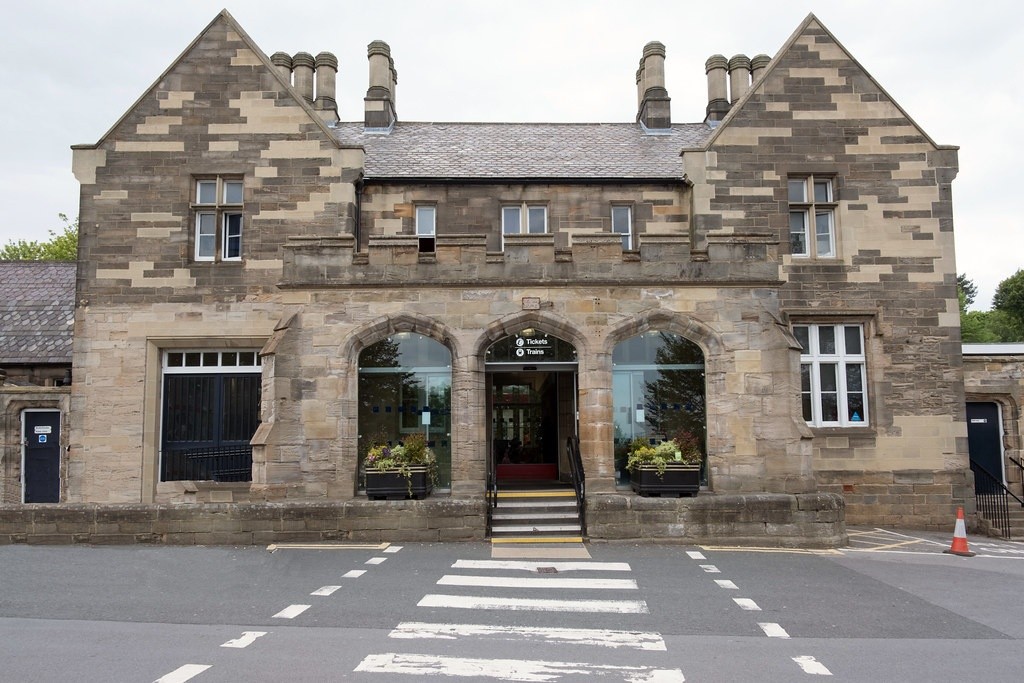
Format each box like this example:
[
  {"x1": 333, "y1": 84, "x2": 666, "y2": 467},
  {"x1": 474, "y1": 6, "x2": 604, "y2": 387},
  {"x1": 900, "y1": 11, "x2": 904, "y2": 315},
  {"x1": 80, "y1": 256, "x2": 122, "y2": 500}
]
[{"x1": 362, "y1": 428, "x2": 443, "y2": 497}]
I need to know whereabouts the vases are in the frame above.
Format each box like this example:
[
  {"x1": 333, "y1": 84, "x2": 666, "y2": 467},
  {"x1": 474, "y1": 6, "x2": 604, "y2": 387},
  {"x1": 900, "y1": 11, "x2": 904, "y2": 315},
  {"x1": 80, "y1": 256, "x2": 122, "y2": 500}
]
[{"x1": 364, "y1": 461, "x2": 433, "y2": 500}]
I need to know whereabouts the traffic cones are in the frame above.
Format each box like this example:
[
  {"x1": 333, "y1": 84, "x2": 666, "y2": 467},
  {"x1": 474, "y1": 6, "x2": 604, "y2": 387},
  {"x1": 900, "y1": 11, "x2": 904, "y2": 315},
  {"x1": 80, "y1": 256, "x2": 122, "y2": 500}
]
[{"x1": 942, "y1": 506, "x2": 976, "y2": 557}]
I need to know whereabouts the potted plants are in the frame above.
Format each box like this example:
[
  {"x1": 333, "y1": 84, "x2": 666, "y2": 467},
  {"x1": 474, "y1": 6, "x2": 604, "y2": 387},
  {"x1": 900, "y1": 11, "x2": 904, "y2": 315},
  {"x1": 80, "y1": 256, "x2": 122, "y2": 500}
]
[{"x1": 629, "y1": 432, "x2": 704, "y2": 498}]
